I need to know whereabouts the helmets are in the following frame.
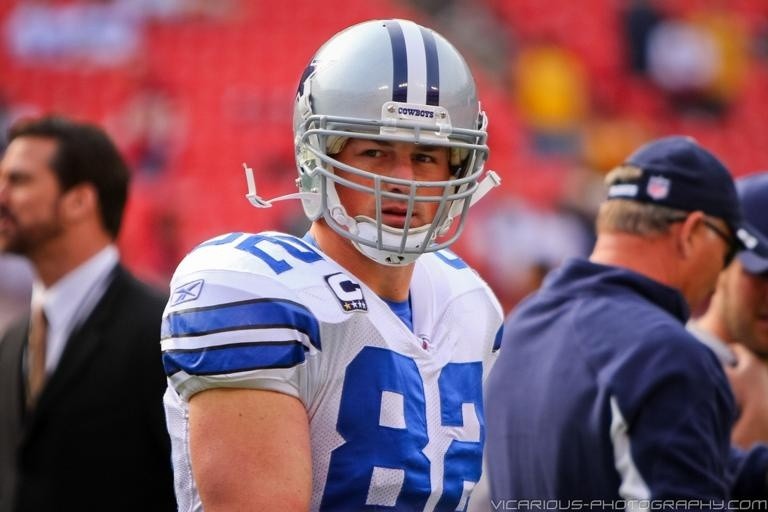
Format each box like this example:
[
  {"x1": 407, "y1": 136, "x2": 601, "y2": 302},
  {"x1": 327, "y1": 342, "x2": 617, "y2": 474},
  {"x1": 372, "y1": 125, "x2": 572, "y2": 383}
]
[{"x1": 293, "y1": 18, "x2": 489, "y2": 226}]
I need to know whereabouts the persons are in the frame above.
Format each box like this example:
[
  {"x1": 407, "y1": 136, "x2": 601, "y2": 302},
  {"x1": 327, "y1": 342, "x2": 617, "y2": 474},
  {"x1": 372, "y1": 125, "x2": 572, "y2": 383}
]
[
  {"x1": 482, "y1": 135, "x2": 768, "y2": 512},
  {"x1": 681, "y1": 170, "x2": 768, "y2": 452},
  {"x1": 160, "y1": 16, "x2": 504, "y2": 512},
  {"x1": 1, "y1": 114, "x2": 181, "y2": 511}
]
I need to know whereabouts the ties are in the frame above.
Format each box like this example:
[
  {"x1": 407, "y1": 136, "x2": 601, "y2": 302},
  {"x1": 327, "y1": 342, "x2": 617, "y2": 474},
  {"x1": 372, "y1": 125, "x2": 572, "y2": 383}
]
[{"x1": 23, "y1": 308, "x2": 47, "y2": 420}]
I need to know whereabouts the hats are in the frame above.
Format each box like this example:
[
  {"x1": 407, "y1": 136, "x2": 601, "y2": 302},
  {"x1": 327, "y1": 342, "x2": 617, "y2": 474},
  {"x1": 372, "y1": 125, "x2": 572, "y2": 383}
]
[
  {"x1": 606, "y1": 135, "x2": 768, "y2": 255},
  {"x1": 732, "y1": 171, "x2": 768, "y2": 275}
]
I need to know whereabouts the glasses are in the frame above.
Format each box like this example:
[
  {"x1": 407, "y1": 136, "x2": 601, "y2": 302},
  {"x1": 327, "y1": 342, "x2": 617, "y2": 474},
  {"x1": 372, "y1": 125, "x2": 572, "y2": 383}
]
[{"x1": 665, "y1": 213, "x2": 741, "y2": 268}]
